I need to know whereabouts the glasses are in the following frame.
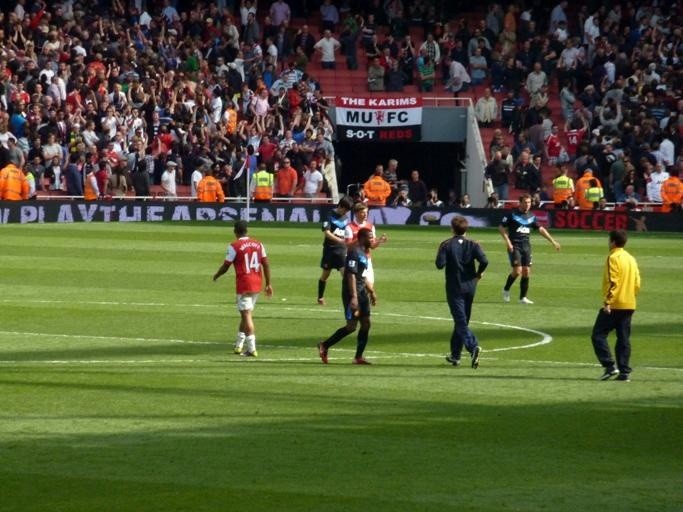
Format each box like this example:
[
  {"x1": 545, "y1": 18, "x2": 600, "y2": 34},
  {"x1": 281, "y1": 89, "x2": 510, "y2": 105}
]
[{"x1": 283, "y1": 162, "x2": 290, "y2": 164}]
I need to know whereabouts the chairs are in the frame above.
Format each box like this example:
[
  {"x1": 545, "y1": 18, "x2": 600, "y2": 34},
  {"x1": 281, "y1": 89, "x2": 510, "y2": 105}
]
[
  {"x1": 272, "y1": 17, "x2": 564, "y2": 126},
  {"x1": 126, "y1": 183, "x2": 192, "y2": 196},
  {"x1": 479, "y1": 126, "x2": 581, "y2": 209},
  {"x1": 36, "y1": 189, "x2": 68, "y2": 196},
  {"x1": 295, "y1": 187, "x2": 328, "y2": 197}
]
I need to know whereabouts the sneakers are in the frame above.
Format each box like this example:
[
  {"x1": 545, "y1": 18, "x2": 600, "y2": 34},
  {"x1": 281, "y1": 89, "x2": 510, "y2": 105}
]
[
  {"x1": 352, "y1": 358, "x2": 370, "y2": 364},
  {"x1": 503, "y1": 290, "x2": 510, "y2": 302},
  {"x1": 239, "y1": 351, "x2": 257, "y2": 357},
  {"x1": 601, "y1": 370, "x2": 632, "y2": 381},
  {"x1": 317, "y1": 342, "x2": 328, "y2": 364},
  {"x1": 472, "y1": 345, "x2": 482, "y2": 369},
  {"x1": 520, "y1": 297, "x2": 534, "y2": 304},
  {"x1": 233, "y1": 346, "x2": 242, "y2": 353},
  {"x1": 318, "y1": 299, "x2": 325, "y2": 304},
  {"x1": 446, "y1": 357, "x2": 460, "y2": 366}
]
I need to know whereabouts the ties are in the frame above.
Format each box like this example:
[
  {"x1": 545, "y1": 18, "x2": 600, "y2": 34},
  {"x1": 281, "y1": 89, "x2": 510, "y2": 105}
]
[{"x1": 134, "y1": 151, "x2": 139, "y2": 171}]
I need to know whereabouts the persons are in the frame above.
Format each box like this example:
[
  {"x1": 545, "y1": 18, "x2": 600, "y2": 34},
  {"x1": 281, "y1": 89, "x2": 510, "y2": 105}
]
[
  {"x1": 435, "y1": 217, "x2": 488, "y2": 369},
  {"x1": 591, "y1": 229, "x2": 641, "y2": 382},
  {"x1": 213, "y1": 220, "x2": 273, "y2": 357},
  {"x1": 498, "y1": 194, "x2": 561, "y2": 304},
  {"x1": 317, "y1": 227, "x2": 377, "y2": 365},
  {"x1": 345, "y1": 203, "x2": 388, "y2": 290},
  {"x1": 318, "y1": 196, "x2": 354, "y2": 306},
  {"x1": 0, "y1": 1, "x2": 682, "y2": 211}
]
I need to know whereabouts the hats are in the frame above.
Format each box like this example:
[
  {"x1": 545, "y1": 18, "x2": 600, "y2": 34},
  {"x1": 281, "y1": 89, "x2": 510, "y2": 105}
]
[
  {"x1": 167, "y1": 161, "x2": 177, "y2": 166},
  {"x1": 40, "y1": 24, "x2": 49, "y2": 33},
  {"x1": 205, "y1": 18, "x2": 213, "y2": 23}
]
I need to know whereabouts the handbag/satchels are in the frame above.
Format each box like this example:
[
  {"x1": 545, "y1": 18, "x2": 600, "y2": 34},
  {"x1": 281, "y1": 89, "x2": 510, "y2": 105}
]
[
  {"x1": 246, "y1": 102, "x2": 256, "y2": 120},
  {"x1": 559, "y1": 146, "x2": 570, "y2": 164}
]
